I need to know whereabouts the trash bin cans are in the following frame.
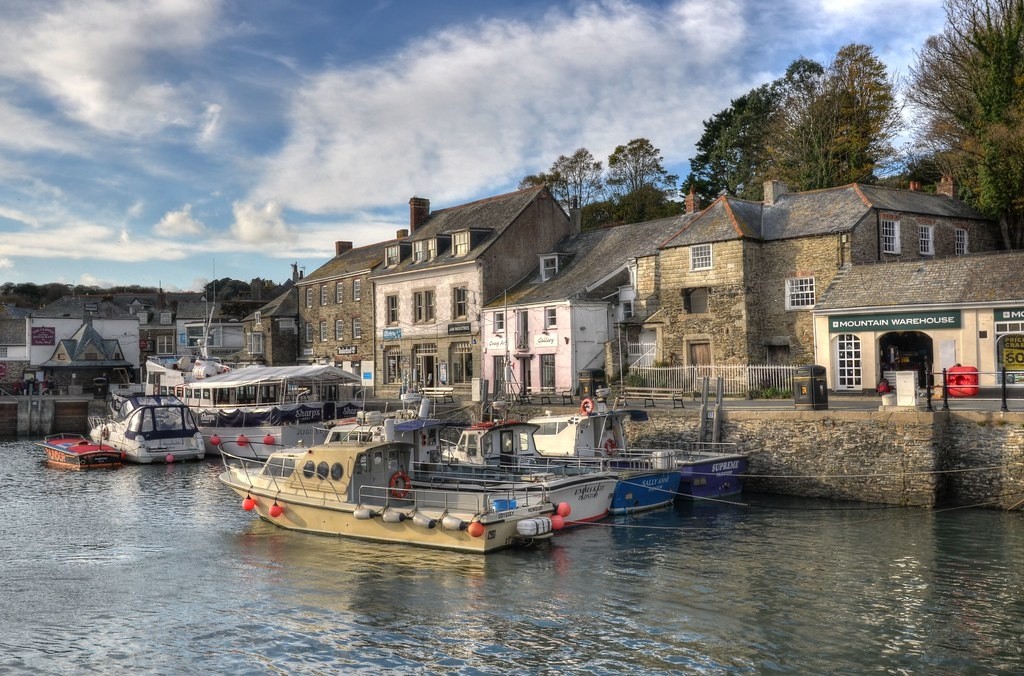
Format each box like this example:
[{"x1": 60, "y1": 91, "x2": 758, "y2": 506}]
[
  {"x1": 578, "y1": 368, "x2": 606, "y2": 403},
  {"x1": 793, "y1": 365, "x2": 828, "y2": 411}
]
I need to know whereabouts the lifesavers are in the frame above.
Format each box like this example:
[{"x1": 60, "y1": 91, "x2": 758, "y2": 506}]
[
  {"x1": 389, "y1": 470, "x2": 411, "y2": 498},
  {"x1": 472, "y1": 421, "x2": 493, "y2": 429},
  {"x1": 499, "y1": 420, "x2": 519, "y2": 425},
  {"x1": 581, "y1": 398, "x2": 594, "y2": 414},
  {"x1": 604, "y1": 439, "x2": 617, "y2": 455}
]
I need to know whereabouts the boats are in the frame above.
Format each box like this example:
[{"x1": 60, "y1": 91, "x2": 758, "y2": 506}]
[
  {"x1": 35, "y1": 433, "x2": 124, "y2": 472},
  {"x1": 110, "y1": 290, "x2": 752, "y2": 530},
  {"x1": 218, "y1": 417, "x2": 555, "y2": 554},
  {"x1": 88, "y1": 394, "x2": 206, "y2": 464}
]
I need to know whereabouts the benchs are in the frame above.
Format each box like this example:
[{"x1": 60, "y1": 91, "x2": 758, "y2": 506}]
[
  {"x1": 541, "y1": 388, "x2": 574, "y2": 405},
  {"x1": 615, "y1": 386, "x2": 685, "y2": 409},
  {"x1": 418, "y1": 387, "x2": 454, "y2": 403}
]
